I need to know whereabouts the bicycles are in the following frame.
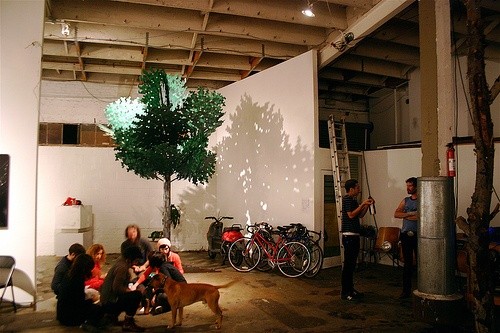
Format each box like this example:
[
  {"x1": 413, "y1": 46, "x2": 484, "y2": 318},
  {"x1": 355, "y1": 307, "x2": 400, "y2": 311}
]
[{"x1": 227, "y1": 221, "x2": 324, "y2": 278}]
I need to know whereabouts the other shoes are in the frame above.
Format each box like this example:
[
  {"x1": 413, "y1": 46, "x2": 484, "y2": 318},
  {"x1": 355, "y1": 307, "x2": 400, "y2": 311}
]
[{"x1": 342, "y1": 294, "x2": 356, "y2": 301}]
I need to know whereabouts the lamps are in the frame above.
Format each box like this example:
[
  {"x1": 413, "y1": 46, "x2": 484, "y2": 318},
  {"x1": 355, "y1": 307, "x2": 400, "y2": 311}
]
[{"x1": 301, "y1": 0, "x2": 319, "y2": 18}]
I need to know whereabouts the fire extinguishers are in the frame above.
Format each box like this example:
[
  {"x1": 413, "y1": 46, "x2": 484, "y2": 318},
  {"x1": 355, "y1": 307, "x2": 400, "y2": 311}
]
[{"x1": 446, "y1": 143, "x2": 455, "y2": 177}]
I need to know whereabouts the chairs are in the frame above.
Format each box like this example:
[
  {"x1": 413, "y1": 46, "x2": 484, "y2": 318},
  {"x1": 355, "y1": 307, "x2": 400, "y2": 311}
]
[
  {"x1": 360, "y1": 225, "x2": 500, "y2": 277},
  {"x1": 0, "y1": 255, "x2": 17, "y2": 314}
]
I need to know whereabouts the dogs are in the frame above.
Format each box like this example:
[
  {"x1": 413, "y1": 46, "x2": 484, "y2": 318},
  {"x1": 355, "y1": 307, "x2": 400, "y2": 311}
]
[{"x1": 149, "y1": 273, "x2": 237, "y2": 329}]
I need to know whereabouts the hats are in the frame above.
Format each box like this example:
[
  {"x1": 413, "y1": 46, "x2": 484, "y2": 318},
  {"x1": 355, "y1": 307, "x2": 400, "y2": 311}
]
[{"x1": 157, "y1": 238, "x2": 171, "y2": 250}]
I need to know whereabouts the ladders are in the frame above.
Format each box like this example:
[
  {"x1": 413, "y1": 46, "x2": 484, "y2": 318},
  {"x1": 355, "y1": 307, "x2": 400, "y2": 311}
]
[{"x1": 328, "y1": 114, "x2": 360, "y2": 272}]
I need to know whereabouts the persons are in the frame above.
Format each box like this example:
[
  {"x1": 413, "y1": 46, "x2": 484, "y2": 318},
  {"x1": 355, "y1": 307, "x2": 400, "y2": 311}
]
[
  {"x1": 136, "y1": 250, "x2": 187, "y2": 314},
  {"x1": 132, "y1": 238, "x2": 184, "y2": 293},
  {"x1": 51, "y1": 242, "x2": 99, "y2": 302},
  {"x1": 99, "y1": 245, "x2": 146, "y2": 332},
  {"x1": 457, "y1": 232, "x2": 500, "y2": 288},
  {"x1": 394, "y1": 177, "x2": 417, "y2": 298},
  {"x1": 84, "y1": 242, "x2": 110, "y2": 291},
  {"x1": 342, "y1": 179, "x2": 374, "y2": 303},
  {"x1": 121, "y1": 224, "x2": 152, "y2": 289},
  {"x1": 55, "y1": 253, "x2": 103, "y2": 332}
]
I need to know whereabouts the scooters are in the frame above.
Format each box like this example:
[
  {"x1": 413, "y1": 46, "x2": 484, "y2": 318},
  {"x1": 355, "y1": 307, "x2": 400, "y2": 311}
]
[{"x1": 205, "y1": 216, "x2": 245, "y2": 266}]
